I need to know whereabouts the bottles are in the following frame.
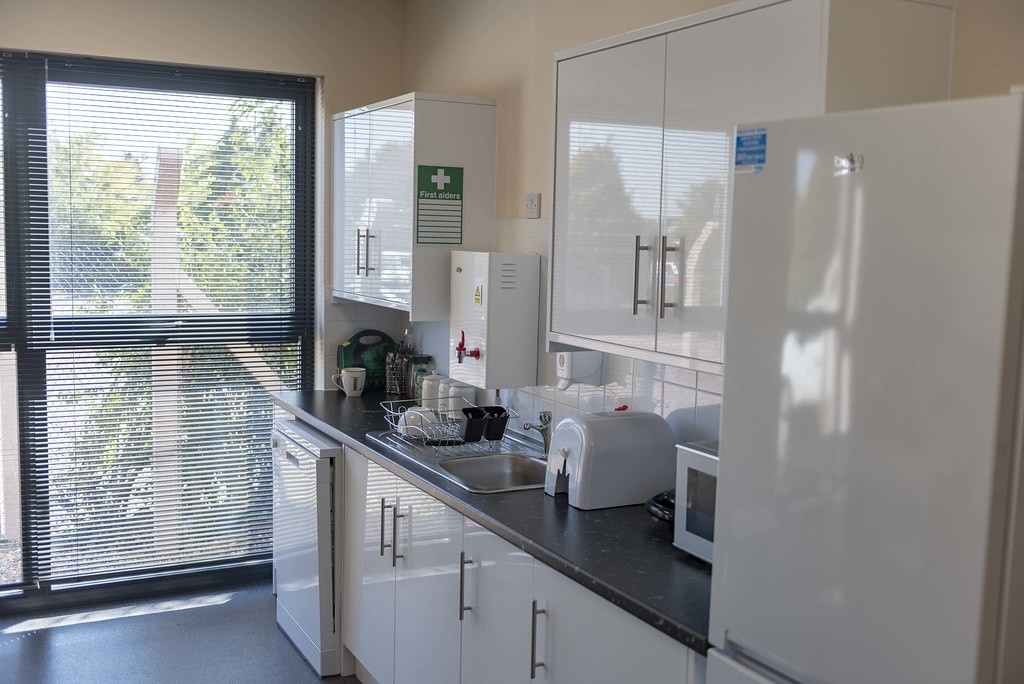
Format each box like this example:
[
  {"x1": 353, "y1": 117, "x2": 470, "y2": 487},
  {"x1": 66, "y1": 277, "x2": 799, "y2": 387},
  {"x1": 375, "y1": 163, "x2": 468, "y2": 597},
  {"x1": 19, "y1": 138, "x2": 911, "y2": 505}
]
[{"x1": 413, "y1": 367, "x2": 427, "y2": 406}]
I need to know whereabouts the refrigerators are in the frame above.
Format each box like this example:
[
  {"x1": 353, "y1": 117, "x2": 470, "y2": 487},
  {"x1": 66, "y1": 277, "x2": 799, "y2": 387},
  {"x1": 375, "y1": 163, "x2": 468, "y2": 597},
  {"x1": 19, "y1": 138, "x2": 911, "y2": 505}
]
[{"x1": 705, "y1": 89, "x2": 1024, "y2": 684}]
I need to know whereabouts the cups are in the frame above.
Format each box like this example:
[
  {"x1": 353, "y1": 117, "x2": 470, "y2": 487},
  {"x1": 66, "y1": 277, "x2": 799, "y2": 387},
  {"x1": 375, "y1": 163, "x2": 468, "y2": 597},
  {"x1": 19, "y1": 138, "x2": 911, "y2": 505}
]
[
  {"x1": 332, "y1": 368, "x2": 366, "y2": 397},
  {"x1": 385, "y1": 362, "x2": 409, "y2": 394},
  {"x1": 421, "y1": 369, "x2": 475, "y2": 419},
  {"x1": 459, "y1": 405, "x2": 510, "y2": 443}
]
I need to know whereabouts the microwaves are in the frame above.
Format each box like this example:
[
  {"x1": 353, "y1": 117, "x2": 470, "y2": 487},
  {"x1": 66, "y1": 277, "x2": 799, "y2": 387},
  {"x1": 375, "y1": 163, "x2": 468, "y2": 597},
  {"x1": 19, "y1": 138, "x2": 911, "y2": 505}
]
[{"x1": 672, "y1": 437, "x2": 720, "y2": 565}]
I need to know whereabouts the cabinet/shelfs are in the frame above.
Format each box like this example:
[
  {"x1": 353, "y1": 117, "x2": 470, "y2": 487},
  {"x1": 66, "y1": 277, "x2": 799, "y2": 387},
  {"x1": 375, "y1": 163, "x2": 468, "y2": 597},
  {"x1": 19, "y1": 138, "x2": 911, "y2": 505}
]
[
  {"x1": 531, "y1": 554, "x2": 707, "y2": 684},
  {"x1": 544, "y1": 0, "x2": 955, "y2": 378},
  {"x1": 332, "y1": 91, "x2": 498, "y2": 323},
  {"x1": 342, "y1": 443, "x2": 463, "y2": 683},
  {"x1": 380, "y1": 396, "x2": 520, "y2": 457},
  {"x1": 460, "y1": 514, "x2": 534, "y2": 684}
]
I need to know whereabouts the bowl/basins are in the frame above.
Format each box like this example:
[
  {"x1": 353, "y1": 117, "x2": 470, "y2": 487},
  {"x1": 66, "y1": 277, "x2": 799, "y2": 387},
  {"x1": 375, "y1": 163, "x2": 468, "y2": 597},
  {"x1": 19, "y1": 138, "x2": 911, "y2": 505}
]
[{"x1": 397, "y1": 406, "x2": 439, "y2": 436}]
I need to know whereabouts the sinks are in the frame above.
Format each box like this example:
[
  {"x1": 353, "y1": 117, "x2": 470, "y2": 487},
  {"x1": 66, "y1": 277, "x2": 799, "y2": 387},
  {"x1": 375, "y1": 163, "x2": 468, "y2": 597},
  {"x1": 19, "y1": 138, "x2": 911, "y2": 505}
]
[{"x1": 437, "y1": 446, "x2": 553, "y2": 494}]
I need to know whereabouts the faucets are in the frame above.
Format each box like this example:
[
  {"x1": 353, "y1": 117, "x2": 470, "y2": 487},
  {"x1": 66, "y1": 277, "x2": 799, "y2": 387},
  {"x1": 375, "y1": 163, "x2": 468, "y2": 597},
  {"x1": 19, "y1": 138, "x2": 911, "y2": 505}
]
[{"x1": 524, "y1": 410, "x2": 551, "y2": 460}]
